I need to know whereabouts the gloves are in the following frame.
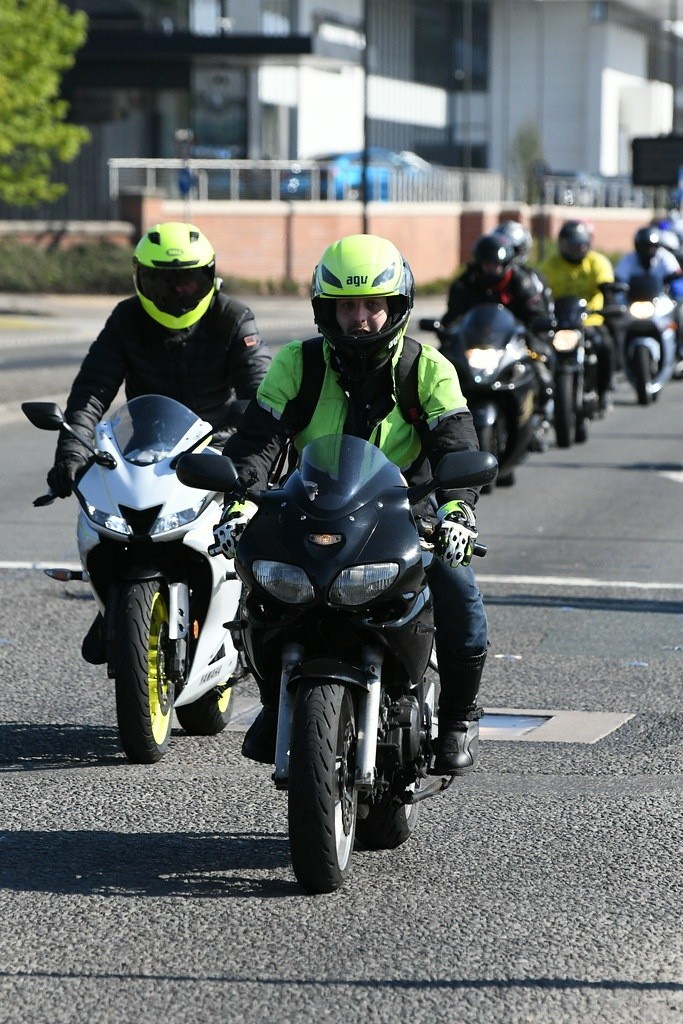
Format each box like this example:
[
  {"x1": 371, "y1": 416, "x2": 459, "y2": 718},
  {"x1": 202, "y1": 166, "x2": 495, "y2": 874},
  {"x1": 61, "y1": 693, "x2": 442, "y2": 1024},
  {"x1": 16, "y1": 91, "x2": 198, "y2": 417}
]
[
  {"x1": 430, "y1": 500, "x2": 478, "y2": 568},
  {"x1": 213, "y1": 498, "x2": 259, "y2": 560},
  {"x1": 46, "y1": 454, "x2": 89, "y2": 499}
]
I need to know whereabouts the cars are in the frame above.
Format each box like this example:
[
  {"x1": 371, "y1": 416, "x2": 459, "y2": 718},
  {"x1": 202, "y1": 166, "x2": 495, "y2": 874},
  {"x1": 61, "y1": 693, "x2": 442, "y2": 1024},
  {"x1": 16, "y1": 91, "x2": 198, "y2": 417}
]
[{"x1": 278, "y1": 145, "x2": 437, "y2": 202}]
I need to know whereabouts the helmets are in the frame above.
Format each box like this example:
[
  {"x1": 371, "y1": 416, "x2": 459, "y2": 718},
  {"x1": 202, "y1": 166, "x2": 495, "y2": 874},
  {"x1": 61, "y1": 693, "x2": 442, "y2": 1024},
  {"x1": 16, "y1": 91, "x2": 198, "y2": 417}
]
[
  {"x1": 311, "y1": 235, "x2": 415, "y2": 371},
  {"x1": 473, "y1": 233, "x2": 516, "y2": 284},
  {"x1": 557, "y1": 220, "x2": 594, "y2": 264},
  {"x1": 634, "y1": 226, "x2": 661, "y2": 252},
  {"x1": 494, "y1": 220, "x2": 533, "y2": 266},
  {"x1": 652, "y1": 216, "x2": 677, "y2": 232},
  {"x1": 132, "y1": 222, "x2": 216, "y2": 328}
]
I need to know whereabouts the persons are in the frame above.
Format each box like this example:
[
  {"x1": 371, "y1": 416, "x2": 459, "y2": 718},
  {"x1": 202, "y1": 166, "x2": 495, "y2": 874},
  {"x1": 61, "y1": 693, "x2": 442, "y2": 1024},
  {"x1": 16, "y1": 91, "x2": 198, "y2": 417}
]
[
  {"x1": 47, "y1": 223, "x2": 271, "y2": 664},
  {"x1": 213, "y1": 234, "x2": 488, "y2": 772},
  {"x1": 439, "y1": 212, "x2": 682, "y2": 451}
]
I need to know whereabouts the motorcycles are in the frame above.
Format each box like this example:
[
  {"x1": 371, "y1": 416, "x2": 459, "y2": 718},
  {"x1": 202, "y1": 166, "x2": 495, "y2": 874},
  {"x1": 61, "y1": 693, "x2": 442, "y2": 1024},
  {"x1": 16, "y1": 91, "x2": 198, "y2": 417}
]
[
  {"x1": 528, "y1": 266, "x2": 683, "y2": 447},
  {"x1": 20, "y1": 394, "x2": 259, "y2": 766},
  {"x1": 417, "y1": 302, "x2": 556, "y2": 496},
  {"x1": 176, "y1": 431, "x2": 499, "y2": 895}
]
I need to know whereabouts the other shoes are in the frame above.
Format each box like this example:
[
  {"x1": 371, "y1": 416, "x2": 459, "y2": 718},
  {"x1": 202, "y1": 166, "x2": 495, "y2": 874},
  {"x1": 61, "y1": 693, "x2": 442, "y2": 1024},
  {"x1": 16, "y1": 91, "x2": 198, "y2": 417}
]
[
  {"x1": 82, "y1": 611, "x2": 112, "y2": 666},
  {"x1": 532, "y1": 427, "x2": 550, "y2": 452},
  {"x1": 593, "y1": 385, "x2": 610, "y2": 411}
]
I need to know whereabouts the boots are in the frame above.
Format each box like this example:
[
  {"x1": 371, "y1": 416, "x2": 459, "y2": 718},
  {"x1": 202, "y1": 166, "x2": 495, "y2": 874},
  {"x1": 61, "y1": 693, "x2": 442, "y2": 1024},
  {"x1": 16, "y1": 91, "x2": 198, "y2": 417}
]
[
  {"x1": 433, "y1": 636, "x2": 485, "y2": 774},
  {"x1": 240, "y1": 672, "x2": 281, "y2": 765}
]
